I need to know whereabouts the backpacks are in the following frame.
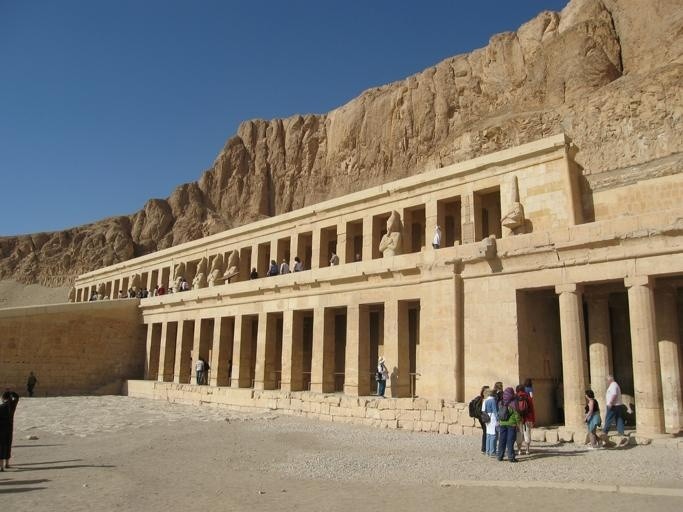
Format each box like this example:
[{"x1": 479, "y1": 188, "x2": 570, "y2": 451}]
[
  {"x1": 498, "y1": 401, "x2": 513, "y2": 421},
  {"x1": 516, "y1": 396, "x2": 531, "y2": 415},
  {"x1": 469, "y1": 397, "x2": 481, "y2": 417}
]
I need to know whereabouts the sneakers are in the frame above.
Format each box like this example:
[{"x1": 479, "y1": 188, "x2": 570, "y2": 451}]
[
  {"x1": 498, "y1": 457, "x2": 517, "y2": 462},
  {"x1": 517, "y1": 450, "x2": 530, "y2": 455}
]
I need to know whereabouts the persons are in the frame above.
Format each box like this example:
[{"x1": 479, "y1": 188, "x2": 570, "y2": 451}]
[
  {"x1": 188, "y1": 356, "x2": 210, "y2": 385},
  {"x1": 378, "y1": 209, "x2": 402, "y2": 259},
  {"x1": 433, "y1": 224, "x2": 442, "y2": 250},
  {"x1": 89, "y1": 283, "x2": 166, "y2": 301},
  {"x1": 249, "y1": 257, "x2": 303, "y2": 280},
  {"x1": 178, "y1": 279, "x2": 183, "y2": 292},
  {"x1": 329, "y1": 251, "x2": 339, "y2": 267},
  {"x1": 583, "y1": 389, "x2": 602, "y2": 448},
  {"x1": 377, "y1": 356, "x2": 389, "y2": 398},
  {"x1": 0, "y1": 391, "x2": 19, "y2": 472},
  {"x1": 597, "y1": 375, "x2": 625, "y2": 436},
  {"x1": 169, "y1": 262, "x2": 184, "y2": 294},
  {"x1": 181, "y1": 278, "x2": 190, "y2": 291},
  {"x1": 478, "y1": 378, "x2": 536, "y2": 463},
  {"x1": 192, "y1": 250, "x2": 239, "y2": 290},
  {"x1": 27, "y1": 371, "x2": 37, "y2": 397}
]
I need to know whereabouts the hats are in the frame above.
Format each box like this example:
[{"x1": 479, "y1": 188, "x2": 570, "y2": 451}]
[{"x1": 379, "y1": 357, "x2": 384, "y2": 363}]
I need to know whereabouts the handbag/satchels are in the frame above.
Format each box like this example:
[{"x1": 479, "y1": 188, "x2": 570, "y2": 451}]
[
  {"x1": 375, "y1": 372, "x2": 382, "y2": 381},
  {"x1": 481, "y1": 411, "x2": 490, "y2": 423}
]
